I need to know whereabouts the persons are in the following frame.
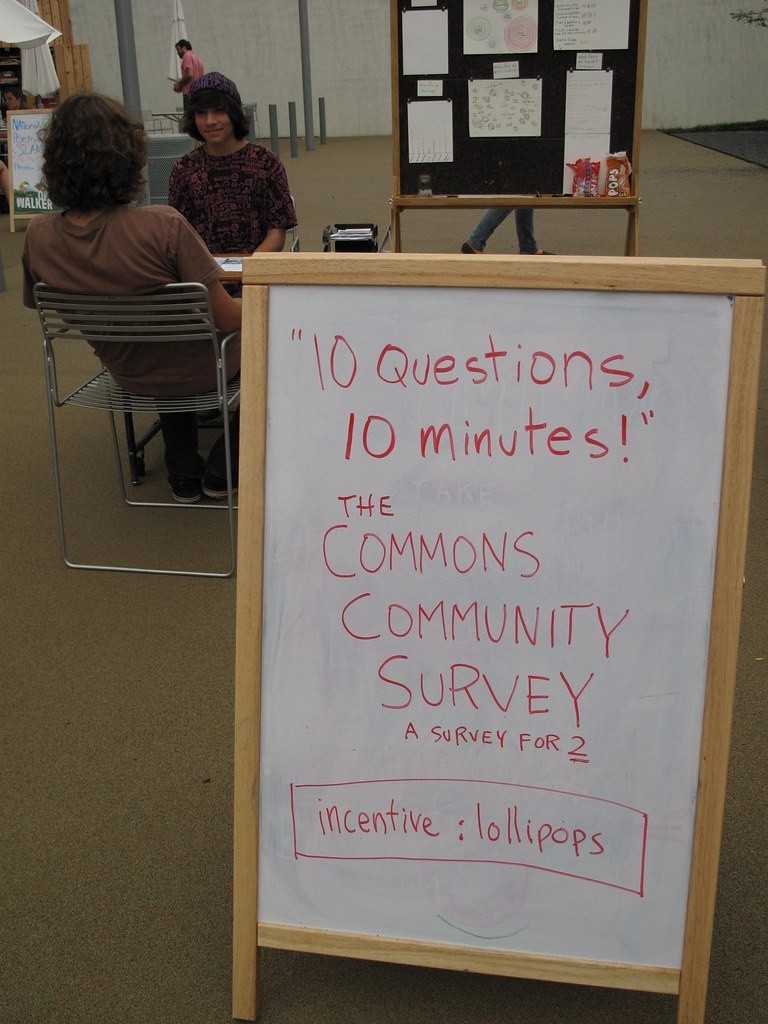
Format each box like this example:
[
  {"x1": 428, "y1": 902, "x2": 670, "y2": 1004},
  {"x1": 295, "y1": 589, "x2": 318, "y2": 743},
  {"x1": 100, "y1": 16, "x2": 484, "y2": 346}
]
[
  {"x1": 0, "y1": 86, "x2": 36, "y2": 206},
  {"x1": 174, "y1": 39, "x2": 204, "y2": 114},
  {"x1": 24, "y1": 71, "x2": 298, "y2": 503},
  {"x1": 461, "y1": 208, "x2": 556, "y2": 255}
]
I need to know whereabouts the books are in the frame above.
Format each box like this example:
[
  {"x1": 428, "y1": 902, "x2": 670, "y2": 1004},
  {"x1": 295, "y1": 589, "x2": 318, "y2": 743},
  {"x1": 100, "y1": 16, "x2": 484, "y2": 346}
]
[{"x1": 330, "y1": 228, "x2": 373, "y2": 241}]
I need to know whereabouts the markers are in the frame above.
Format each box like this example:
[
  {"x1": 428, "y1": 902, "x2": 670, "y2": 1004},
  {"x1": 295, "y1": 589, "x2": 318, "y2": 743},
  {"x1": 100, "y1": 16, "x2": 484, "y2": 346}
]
[{"x1": 433, "y1": 193, "x2": 458, "y2": 199}]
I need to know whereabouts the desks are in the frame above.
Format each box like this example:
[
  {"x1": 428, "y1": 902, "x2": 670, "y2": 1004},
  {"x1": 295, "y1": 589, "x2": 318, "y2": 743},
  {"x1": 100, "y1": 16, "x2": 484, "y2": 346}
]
[
  {"x1": 123, "y1": 253, "x2": 253, "y2": 480},
  {"x1": 153, "y1": 111, "x2": 184, "y2": 124}
]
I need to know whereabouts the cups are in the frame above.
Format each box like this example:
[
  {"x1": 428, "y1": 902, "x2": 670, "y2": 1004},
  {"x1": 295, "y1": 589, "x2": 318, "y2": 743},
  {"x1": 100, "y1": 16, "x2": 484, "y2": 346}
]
[{"x1": 418, "y1": 176, "x2": 432, "y2": 197}]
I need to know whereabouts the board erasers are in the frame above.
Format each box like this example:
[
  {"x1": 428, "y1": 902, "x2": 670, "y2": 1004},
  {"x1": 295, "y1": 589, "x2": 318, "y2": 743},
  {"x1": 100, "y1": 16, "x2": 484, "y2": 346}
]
[{"x1": 418, "y1": 175, "x2": 433, "y2": 197}]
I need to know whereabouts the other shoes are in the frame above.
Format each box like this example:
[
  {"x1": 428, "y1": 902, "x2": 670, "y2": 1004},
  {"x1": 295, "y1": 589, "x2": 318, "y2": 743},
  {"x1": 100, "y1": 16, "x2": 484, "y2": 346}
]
[
  {"x1": 519, "y1": 248, "x2": 555, "y2": 255},
  {"x1": 462, "y1": 242, "x2": 476, "y2": 253},
  {"x1": 168, "y1": 471, "x2": 202, "y2": 504},
  {"x1": 202, "y1": 470, "x2": 237, "y2": 498}
]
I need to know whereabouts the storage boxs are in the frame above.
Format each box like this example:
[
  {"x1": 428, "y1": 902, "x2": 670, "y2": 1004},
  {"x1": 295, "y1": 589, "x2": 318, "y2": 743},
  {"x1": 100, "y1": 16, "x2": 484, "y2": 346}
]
[{"x1": 323, "y1": 223, "x2": 380, "y2": 253}]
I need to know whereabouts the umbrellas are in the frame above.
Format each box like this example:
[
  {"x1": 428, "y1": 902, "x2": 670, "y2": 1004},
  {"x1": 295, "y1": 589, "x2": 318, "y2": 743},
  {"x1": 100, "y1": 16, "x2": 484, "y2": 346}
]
[
  {"x1": 167, "y1": 0, "x2": 188, "y2": 82},
  {"x1": 0, "y1": 0, "x2": 63, "y2": 108}
]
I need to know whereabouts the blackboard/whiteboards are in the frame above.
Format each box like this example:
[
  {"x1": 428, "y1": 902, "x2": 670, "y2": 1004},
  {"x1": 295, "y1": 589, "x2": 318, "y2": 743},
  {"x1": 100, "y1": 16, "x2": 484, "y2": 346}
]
[
  {"x1": 204, "y1": 248, "x2": 758, "y2": 981},
  {"x1": 7, "y1": 107, "x2": 66, "y2": 219},
  {"x1": 391, "y1": 0, "x2": 647, "y2": 207}
]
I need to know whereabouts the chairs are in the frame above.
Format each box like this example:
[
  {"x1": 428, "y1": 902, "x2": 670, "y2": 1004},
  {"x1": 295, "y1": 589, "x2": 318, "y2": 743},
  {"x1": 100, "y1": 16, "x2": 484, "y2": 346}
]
[
  {"x1": 141, "y1": 106, "x2": 198, "y2": 206},
  {"x1": 35, "y1": 195, "x2": 301, "y2": 577}
]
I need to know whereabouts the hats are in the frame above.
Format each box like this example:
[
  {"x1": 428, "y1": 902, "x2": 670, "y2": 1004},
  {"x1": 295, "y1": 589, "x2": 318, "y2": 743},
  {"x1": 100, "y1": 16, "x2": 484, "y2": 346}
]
[{"x1": 189, "y1": 72, "x2": 240, "y2": 105}]
